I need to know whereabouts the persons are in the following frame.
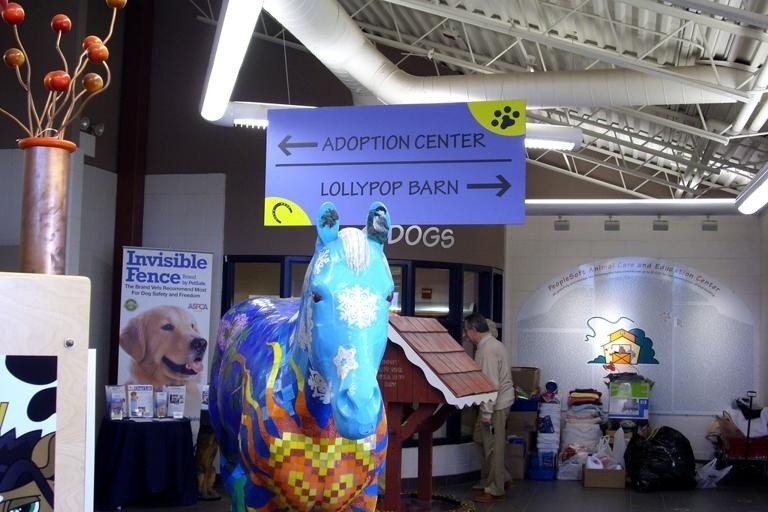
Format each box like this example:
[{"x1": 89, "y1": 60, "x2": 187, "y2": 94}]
[{"x1": 462, "y1": 311, "x2": 518, "y2": 505}]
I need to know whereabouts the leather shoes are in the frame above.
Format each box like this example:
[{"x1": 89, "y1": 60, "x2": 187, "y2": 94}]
[{"x1": 472, "y1": 478, "x2": 512, "y2": 502}]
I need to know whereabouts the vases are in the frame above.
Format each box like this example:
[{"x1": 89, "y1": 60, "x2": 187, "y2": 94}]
[{"x1": 17, "y1": 137, "x2": 77, "y2": 276}]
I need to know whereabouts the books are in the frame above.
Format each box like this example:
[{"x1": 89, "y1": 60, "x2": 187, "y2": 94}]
[{"x1": 104, "y1": 383, "x2": 186, "y2": 424}]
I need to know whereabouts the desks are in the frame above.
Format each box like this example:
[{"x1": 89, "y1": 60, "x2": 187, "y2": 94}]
[{"x1": 94, "y1": 415, "x2": 198, "y2": 512}]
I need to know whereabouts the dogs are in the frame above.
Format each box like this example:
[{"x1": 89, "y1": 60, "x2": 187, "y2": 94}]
[{"x1": 119, "y1": 307, "x2": 208, "y2": 418}]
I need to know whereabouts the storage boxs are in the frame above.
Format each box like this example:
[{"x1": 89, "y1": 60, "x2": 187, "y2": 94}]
[{"x1": 504, "y1": 367, "x2": 649, "y2": 488}]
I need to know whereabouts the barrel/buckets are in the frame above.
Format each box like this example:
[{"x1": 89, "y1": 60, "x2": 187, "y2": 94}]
[{"x1": 585, "y1": 453, "x2": 604, "y2": 470}]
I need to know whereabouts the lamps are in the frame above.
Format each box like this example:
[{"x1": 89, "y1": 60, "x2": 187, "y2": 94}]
[
  {"x1": 553, "y1": 215, "x2": 718, "y2": 231},
  {"x1": 200, "y1": 0, "x2": 583, "y2": 149},
  {"x1": 734, "y1": 162, "x2": 768, "y2": 215}
]
